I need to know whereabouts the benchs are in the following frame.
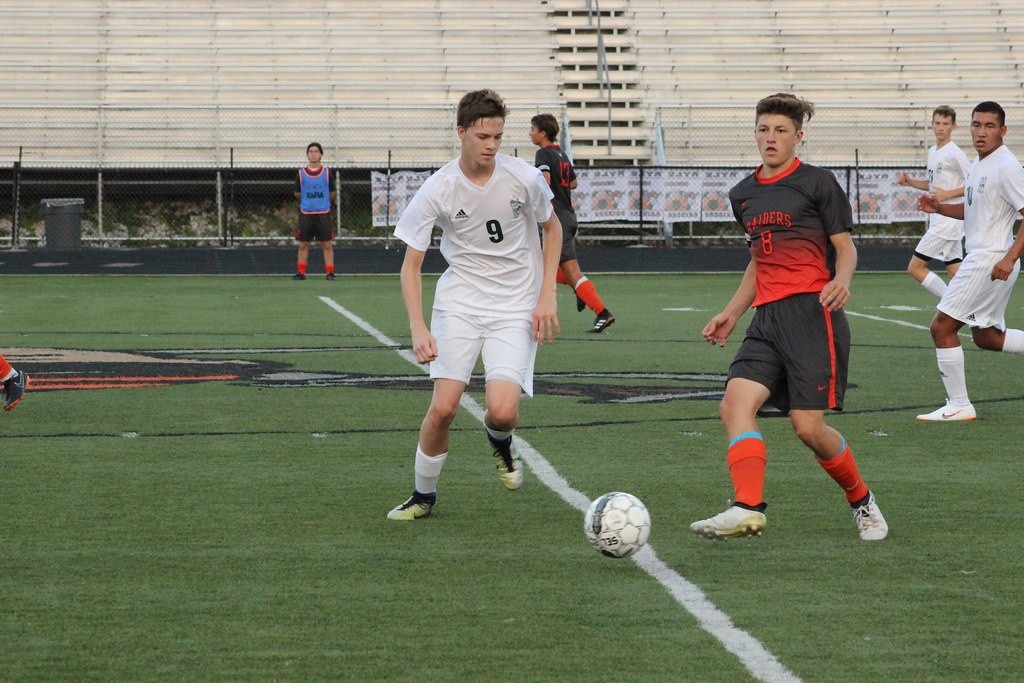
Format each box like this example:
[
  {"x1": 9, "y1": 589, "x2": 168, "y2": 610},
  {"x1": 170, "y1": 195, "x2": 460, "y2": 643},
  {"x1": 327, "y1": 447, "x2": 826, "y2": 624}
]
[{"x1": 0, "y1": 0, "x2": 1024, "y2": 167}]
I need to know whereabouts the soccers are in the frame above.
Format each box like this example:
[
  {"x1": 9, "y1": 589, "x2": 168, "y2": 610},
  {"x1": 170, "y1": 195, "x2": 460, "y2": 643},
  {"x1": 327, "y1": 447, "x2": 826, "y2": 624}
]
[{"x1": 583, "y1": 491, "x2": 652, "y2": 559}]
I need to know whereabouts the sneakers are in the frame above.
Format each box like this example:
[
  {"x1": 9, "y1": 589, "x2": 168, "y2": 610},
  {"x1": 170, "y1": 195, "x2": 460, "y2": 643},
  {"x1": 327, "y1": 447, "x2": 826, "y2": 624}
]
[
  {"x1": 690, "y1": 502, "x2": 768, "y2": 541},
  {"x1": 574, "y1": 289, "x2": 586, "y2": 312},
  {"x1": 3, "y1": 370, "x2": 31, "y2": 410},
  {"x1": 486, "y1": 429, "x2": 523, "y2": 490},
  {"x1": 387, "y1": 490, "x2": 436, "y2": 521},
  {"x1": 326, "y1": 273, "x2": 337, "y2": 280},
  {"x1": 850, "y1": 489, "x2": 888, "y2": 541},
  {"x1": 292, "y1": 273, "x2": 306, "y2": 280},
  {"x1": 588, "y1": 308, "x2": 615, "y2": 333},
  {"x1": 916, "y1": 398, "x2": 976, "y2": 420}
]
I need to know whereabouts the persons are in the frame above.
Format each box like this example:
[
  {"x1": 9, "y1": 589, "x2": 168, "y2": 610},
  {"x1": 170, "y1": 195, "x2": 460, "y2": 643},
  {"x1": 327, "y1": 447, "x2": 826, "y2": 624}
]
[
  {"x1": 689, "y1": 93, "x2": 890, "y2": 542},
  {"x1": 896, "y1": 105, "x2": 972, "y2": 299},
  {"x1": 0, "y1": 352, "x2": 32, "y2": 411},
  {"x1": 387, "y1": 87, "x2": 565, "y2": 522},
  {"x1": 292, "y1": 142, "x2": 338, "y2": 282},
  {"x1": 528, "y1": 113, "x2": 615, "y2": 334},
  {"x1": 913, "y1": 102, "x2": 1024, "y2": 421}
]
